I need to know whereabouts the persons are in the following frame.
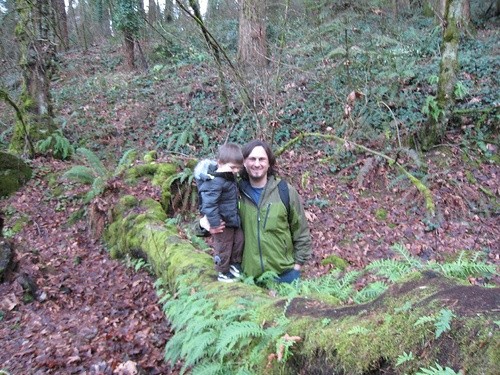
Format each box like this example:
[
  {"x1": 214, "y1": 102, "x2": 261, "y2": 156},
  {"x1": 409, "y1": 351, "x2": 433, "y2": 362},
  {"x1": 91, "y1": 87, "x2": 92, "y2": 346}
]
[
  {"x1": 194, "y1": 142, "x2": 245, "y2": 284},
  {"x1": 191, "y1": 139, "x2": 310, "y2": 294}
]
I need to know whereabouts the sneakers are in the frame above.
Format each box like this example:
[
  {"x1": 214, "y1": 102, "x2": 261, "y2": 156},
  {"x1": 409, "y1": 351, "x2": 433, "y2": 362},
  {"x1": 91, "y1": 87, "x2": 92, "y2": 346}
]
[
  {"x1": 227, "y1": 263, "x2": 246, "y2": 280},
  {"x1": 217, "y1": 271, "x2": 240, "y2": 284}
]
